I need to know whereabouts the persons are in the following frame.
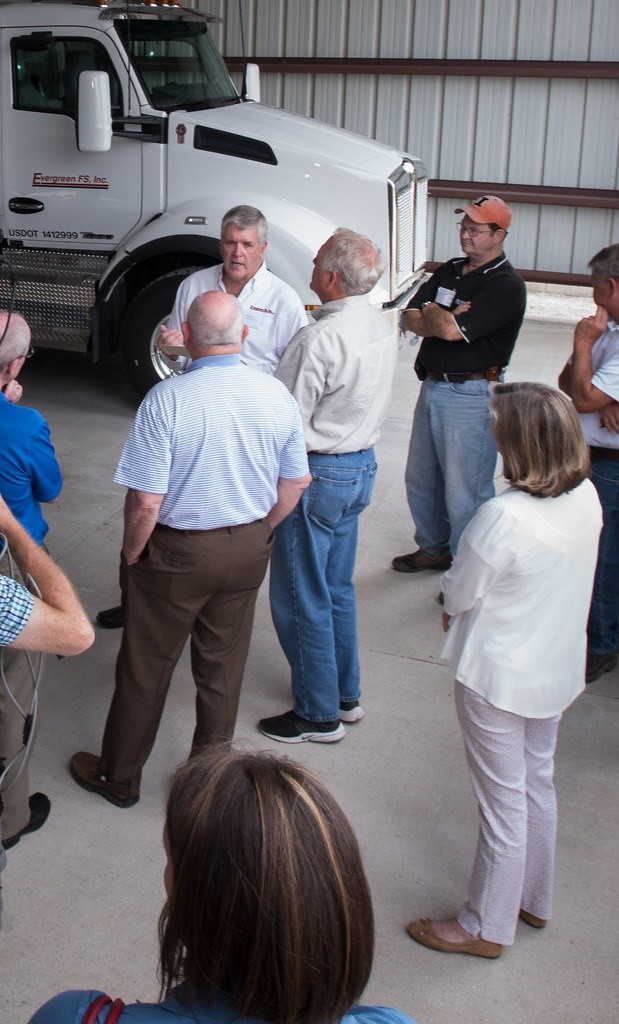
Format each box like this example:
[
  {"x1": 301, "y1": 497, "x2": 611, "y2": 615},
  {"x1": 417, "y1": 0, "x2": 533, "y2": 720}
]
[
  {"x1": 557, "y1": 242, "x2": 618, "y2": 684},
  {"x1": 406, "y1": 380, "x2": 603, "y2": 958},
  {"x1": 391, "y1": 195, "x2": 528, "y2": 577},
  {"x1": 0, "y1": 499, "x2": 95, "y2": 657},
  {"x1": 68, "y1": 292, "x2": 313, "y2": 809},
  {"x1": 258, "y1": 228, "x2": 397, "y2": 742},
  {"x1": 95, "y1": 205, "x2": 309, "y2": 632},
  {"x1": 1, "y1": 312, "x2": 62, "y2": 849},
  {"x1": 24, "y1": 753, "x2": 416, "y2": 1024}
]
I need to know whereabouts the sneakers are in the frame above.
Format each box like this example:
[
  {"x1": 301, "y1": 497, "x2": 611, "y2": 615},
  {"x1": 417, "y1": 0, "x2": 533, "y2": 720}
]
[
  {"x1": 70, "y1": 751, "x2": 140, "y2": 806},
  {"x1": 584, "y1": 653, "x2": 619, "y2": 683},
  {"x1": 337, "y1": 698, "x2": 364, "y2": 722},
  {"x1": 259, "y1": 709, "x2": 345, "y2": 744},
  {"x1": 392, "y1": 551, "x2": 454, "y2": 572}
]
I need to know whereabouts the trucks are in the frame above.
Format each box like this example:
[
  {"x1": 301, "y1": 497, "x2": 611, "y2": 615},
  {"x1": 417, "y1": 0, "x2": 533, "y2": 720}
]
[{"x1": 1, "y1": 0, "x2": 435, "y2": 413}]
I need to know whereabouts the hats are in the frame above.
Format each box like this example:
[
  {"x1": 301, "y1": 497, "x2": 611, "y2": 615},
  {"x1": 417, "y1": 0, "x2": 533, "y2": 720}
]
[{"x1": 455, "y1": 196, "x2": 512, "y2": 234}]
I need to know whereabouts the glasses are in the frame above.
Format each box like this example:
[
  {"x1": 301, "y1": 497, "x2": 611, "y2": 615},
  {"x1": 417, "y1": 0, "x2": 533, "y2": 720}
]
[
  {"x1": 456, "y1": 222, "x2": 496, "y2": 237},
  {"x1": 5, "y1": 345, "x2": 35, "y2": 375}
]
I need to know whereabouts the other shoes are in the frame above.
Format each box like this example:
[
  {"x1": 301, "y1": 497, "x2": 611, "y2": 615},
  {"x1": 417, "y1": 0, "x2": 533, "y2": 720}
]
[
  {"x1": 96, "y1": 606, "x2": 128, "y2": 629},
  {"x1": 519, "y1": 909, "x2": 545, "y2": 929},
  {"x1": 408, "y1": 918, "x2": 504, "y2": 961}
]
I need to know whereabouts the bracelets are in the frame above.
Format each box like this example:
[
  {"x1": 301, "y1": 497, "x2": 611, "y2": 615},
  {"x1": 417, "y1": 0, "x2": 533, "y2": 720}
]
[{"x1": 421, "y1": 301, "x2": 432, "y2": 309}]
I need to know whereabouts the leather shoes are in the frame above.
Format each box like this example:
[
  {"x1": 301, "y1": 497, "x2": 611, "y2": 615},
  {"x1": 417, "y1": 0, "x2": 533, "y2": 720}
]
[{"x1": 2, "y1": 792, "x2": 52, "y2": 850}]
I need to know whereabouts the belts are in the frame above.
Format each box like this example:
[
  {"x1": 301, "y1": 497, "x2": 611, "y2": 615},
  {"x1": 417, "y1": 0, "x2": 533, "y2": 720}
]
[
  {"x1": 428, "y1": 367, "x2": 504, "y2": 385},
  {"x1": 589, "y1": 444, "x2": 619, "y2": 458}
]
